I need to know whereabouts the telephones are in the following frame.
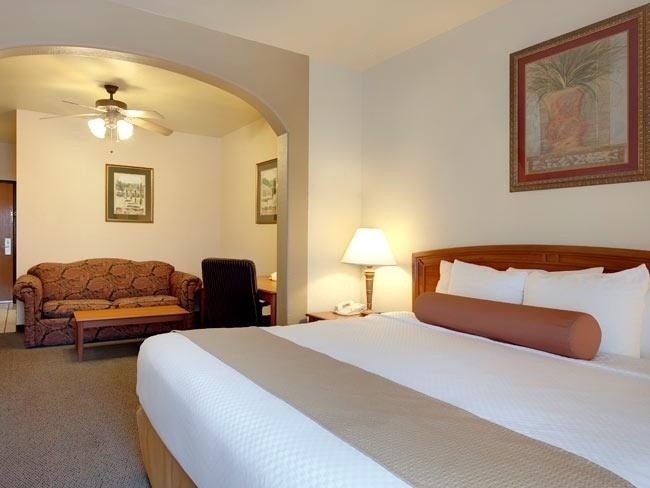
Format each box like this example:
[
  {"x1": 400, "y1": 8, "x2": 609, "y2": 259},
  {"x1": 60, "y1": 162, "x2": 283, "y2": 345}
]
[{"x1": 332, "y1": 301, "x2": 367, "y2": 316}]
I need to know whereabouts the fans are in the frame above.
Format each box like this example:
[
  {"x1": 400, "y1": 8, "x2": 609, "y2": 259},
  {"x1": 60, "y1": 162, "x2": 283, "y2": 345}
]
[{"x1": 39, "y1": 85, "x2": 173, "y2": 137}]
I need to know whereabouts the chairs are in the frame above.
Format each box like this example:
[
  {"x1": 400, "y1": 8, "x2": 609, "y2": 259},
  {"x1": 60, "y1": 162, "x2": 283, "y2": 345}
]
[{"x1": 199, "y1": 256, "x2": 266, "y2": 328}]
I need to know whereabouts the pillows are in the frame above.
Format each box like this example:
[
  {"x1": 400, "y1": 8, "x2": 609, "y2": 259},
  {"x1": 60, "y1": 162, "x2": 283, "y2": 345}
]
[{"x1": 415, "y1": 257, "x2": 650, "y2": 364}]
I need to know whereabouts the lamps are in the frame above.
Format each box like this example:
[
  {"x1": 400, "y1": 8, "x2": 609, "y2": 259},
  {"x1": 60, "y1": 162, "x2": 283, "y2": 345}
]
[
  {"x1": 87, "y1": 112, "x2": 134, "y2": 142},
  {"x1": 340, "y1": 228, "x2": 397, "y2": 316}
]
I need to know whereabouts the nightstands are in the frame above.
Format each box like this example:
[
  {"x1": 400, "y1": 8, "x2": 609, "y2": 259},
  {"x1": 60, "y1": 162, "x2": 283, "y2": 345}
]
[{"x1": 304, "y1": 310, "x2": 383, "y2": 322}]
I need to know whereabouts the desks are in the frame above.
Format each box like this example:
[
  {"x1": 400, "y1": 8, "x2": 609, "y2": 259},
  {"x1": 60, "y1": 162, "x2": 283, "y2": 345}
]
[{"x1": 255, "y1": 274, "x2": 278, "y2": 327}]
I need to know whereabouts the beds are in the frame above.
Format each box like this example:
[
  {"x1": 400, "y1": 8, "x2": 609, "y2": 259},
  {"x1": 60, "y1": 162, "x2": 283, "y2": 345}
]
[{"x1": 134, "y1": 243, "x2": 650, "y2": 488}]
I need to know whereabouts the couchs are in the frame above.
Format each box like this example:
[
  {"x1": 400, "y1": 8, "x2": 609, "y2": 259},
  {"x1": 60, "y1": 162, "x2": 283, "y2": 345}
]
[{"x1": 14, "y1": 258, "x2": 204, "y2": 351}]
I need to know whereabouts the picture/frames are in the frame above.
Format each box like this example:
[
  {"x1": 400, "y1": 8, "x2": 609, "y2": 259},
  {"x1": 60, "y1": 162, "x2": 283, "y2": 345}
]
[
  {"x1": 255, "y1": 158, "x2": 279, "y2": 225},
  {"x1": 506, "y1": 3, "x2": 650, "y2": 193},
  {"x1": 104, "y1": 163, "x2": 155, "y2": 224}
]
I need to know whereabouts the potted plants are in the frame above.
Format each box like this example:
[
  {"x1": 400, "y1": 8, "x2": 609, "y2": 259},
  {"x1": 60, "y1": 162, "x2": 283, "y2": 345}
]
[{"x1": 527, "y1": 37, "x2": 628, "y2": 154}]
